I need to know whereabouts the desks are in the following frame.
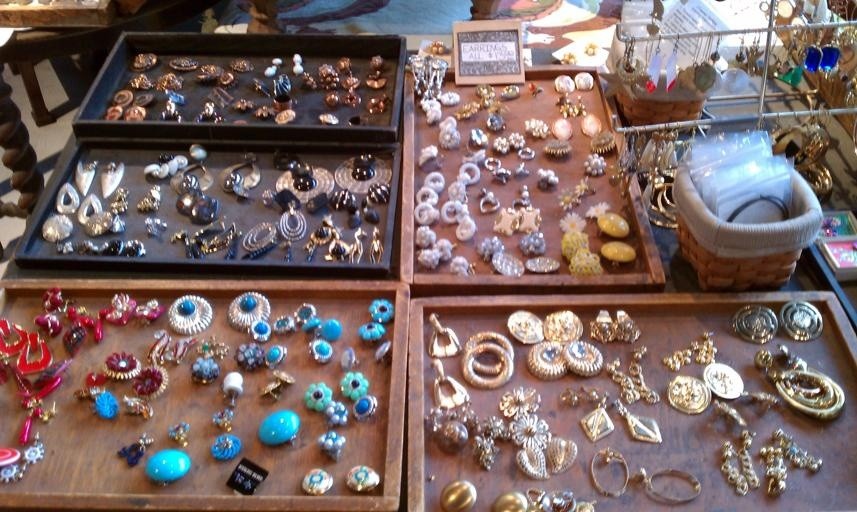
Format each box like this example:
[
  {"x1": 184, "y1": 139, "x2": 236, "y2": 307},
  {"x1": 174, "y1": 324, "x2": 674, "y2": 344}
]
[{"x1": 1, "y1": 1, "x2": 280, "y2": 213}]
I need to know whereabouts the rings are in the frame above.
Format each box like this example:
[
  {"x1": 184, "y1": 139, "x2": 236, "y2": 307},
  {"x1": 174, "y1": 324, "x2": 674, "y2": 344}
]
[
  {"x1": 587, "y1": 446, "x2": 632, "y2": 497},
  {"x1": 631, "y1": 466, "x2": 702, "y2": 506}
]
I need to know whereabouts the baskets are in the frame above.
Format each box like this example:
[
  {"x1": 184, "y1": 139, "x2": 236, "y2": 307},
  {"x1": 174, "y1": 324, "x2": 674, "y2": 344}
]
[
  {"x1": 616, "y1": 81, "x2": 707, "y2": 128},
  {"x1": 674, "y1": 167, "x2": 822, "y2": 293}
]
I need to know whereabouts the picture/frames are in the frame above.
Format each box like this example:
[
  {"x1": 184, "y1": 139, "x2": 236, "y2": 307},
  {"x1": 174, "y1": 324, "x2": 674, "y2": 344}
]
[
  {"x1": 452, "y1": 18, "x2": 525, "y2": 85},
  {"x1": 814, "y1": 209, "x2": 857, "y2": 282}
]
